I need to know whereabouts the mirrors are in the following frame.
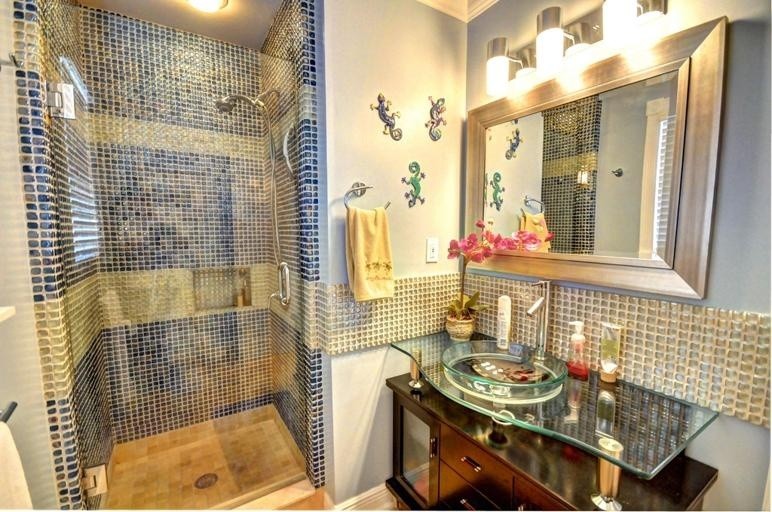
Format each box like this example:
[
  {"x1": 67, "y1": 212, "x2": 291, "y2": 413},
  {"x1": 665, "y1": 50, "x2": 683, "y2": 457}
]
[{"x1": 463, "y1": 16, "x2": 726, "y2": 301}]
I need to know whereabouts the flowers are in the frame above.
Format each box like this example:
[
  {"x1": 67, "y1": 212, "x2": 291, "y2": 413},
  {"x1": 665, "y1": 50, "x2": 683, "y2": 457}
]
[
  {"x1": 475, "y1": 214, "x2": 554, "y2": 251},
  {"x1": 445, "y1": 233, "x2": 490, "y2": 320}
]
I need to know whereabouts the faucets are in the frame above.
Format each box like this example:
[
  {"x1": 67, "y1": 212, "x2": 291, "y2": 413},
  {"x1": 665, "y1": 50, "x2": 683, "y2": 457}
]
[{"x1": 526, "y1": 278, "x2": 550, "y2": 359}]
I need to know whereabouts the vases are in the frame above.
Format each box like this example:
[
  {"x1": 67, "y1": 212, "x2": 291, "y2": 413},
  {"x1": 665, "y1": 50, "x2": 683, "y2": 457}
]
[{"x1": 445, "y1": 315, "x2": 476, "y2": 341}]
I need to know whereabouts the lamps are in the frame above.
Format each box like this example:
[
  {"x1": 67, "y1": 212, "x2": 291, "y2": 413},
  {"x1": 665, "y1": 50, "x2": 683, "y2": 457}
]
[{"x1": 485, "y1": 0, "x2": 668, "y2": 68}]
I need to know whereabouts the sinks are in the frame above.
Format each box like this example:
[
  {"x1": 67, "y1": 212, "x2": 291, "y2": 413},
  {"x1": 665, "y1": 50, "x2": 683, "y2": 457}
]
[{"x1": 441, "y1": 339, "x2": 567, "y2": 402}]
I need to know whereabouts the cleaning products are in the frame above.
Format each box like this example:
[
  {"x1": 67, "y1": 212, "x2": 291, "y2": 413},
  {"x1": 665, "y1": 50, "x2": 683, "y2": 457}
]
[{"x1": 497, "y1": 294, "x2": 512, "y2": 349}]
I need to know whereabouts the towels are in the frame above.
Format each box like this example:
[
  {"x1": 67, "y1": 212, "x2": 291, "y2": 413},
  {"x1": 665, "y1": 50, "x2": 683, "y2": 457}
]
[
  {"x1": 345, "y1": 206, "x2": 395, "y2": 302},
  {"x1": 520, "y1": 214, "x2": 551, "y2": 252}
]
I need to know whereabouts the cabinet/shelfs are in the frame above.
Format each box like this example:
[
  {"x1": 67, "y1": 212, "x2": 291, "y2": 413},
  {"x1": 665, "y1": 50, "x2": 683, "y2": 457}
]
[{"x1": 386, "y1": 392, "x2": 571, "y2": 511}]
